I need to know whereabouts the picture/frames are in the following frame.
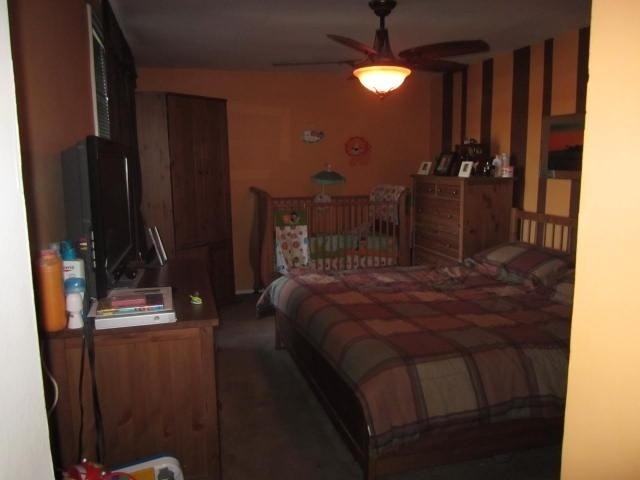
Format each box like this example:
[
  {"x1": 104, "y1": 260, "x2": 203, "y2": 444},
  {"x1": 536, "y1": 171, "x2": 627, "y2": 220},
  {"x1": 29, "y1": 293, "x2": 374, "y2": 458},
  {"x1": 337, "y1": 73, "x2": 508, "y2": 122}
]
[
  {"x1": 418, "y1": 142, "x2": 484, "y2": 178},
  {"x1": 541, "y1": 112, "x2": 587, "y2": 180}
]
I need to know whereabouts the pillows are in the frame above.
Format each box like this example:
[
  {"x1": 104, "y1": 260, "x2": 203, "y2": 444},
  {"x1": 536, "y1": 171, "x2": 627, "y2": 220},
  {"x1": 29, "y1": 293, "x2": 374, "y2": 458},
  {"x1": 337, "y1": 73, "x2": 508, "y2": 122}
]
[{"x1": 465, "y1": 241, "x2": 574, "y2": 307}]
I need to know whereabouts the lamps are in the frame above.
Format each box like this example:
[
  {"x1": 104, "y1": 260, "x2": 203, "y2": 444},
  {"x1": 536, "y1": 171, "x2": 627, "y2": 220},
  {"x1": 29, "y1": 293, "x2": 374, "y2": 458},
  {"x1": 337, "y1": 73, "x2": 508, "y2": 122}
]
[
  {"x1": 308, "y1": 164, "x2": 348, "y2": 203},
  {"x1": 354, "y1": 0, "x2": 411, "y2": 96}
]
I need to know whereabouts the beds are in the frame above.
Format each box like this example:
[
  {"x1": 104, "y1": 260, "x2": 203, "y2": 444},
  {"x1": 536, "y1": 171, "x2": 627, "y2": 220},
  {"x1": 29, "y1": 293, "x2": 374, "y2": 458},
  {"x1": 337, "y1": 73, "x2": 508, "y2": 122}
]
[
  {"x1": 250, "y1": 182, "x2": 405, "y2": 319},
  {"x1": 249, "y1": 208, "x2": 581, "y2": 480}
]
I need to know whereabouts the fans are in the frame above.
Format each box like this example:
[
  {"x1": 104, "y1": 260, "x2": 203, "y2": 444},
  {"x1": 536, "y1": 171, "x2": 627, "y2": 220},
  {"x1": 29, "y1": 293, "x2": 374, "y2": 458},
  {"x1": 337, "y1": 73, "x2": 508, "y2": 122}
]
[{"x1": 272, "y1": 0, "x2": 492, "y2": 87}]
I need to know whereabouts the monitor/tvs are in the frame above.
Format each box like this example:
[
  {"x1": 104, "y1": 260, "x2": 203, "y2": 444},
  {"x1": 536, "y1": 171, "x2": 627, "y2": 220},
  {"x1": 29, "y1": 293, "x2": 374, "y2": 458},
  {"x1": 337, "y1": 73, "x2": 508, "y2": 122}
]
[{"x1": 59, "y1": 135, "x2": 136, "y2": 298}]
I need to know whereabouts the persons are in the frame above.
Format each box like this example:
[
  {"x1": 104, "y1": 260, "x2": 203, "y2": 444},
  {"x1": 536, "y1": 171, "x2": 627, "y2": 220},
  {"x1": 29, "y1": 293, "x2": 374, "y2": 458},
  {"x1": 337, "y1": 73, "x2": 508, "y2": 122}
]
[{"x1": 288, "y1": 212, "x2": 301, "y2": 225}]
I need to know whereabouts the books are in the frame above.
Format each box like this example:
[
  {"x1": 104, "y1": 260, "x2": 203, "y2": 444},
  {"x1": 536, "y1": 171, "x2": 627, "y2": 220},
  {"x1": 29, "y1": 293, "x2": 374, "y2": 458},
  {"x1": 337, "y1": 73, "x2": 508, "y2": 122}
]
[{"x1": 86, "y1": 285, "x2": 177, "y2": 330}]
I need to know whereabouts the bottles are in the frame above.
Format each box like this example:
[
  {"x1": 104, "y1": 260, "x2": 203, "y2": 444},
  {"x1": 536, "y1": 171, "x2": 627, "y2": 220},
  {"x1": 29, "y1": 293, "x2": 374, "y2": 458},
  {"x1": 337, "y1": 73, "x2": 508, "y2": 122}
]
[
  {"x1": 482, "y1": 152, "x2": 513, "y2": 178},
  {"x1": 36, "y1": 240, "x2": 87, "y2": 331}
]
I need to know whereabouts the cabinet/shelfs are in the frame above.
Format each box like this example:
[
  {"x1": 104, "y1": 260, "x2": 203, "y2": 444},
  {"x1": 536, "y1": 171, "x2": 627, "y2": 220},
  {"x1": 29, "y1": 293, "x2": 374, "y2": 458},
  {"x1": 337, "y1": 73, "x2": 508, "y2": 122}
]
[
  {"x1": 141, "y1": 92, "x2": 234, "y2": 244},
  {"x1": 159, "y1": 246, "x2": 235, "y2": 298},
  {"x1": 97, "y1": 262, "x2": 218, "y2": 480},
  {"x1": 410, "y1": 171, "x2": 512, "y2": 266}
]
